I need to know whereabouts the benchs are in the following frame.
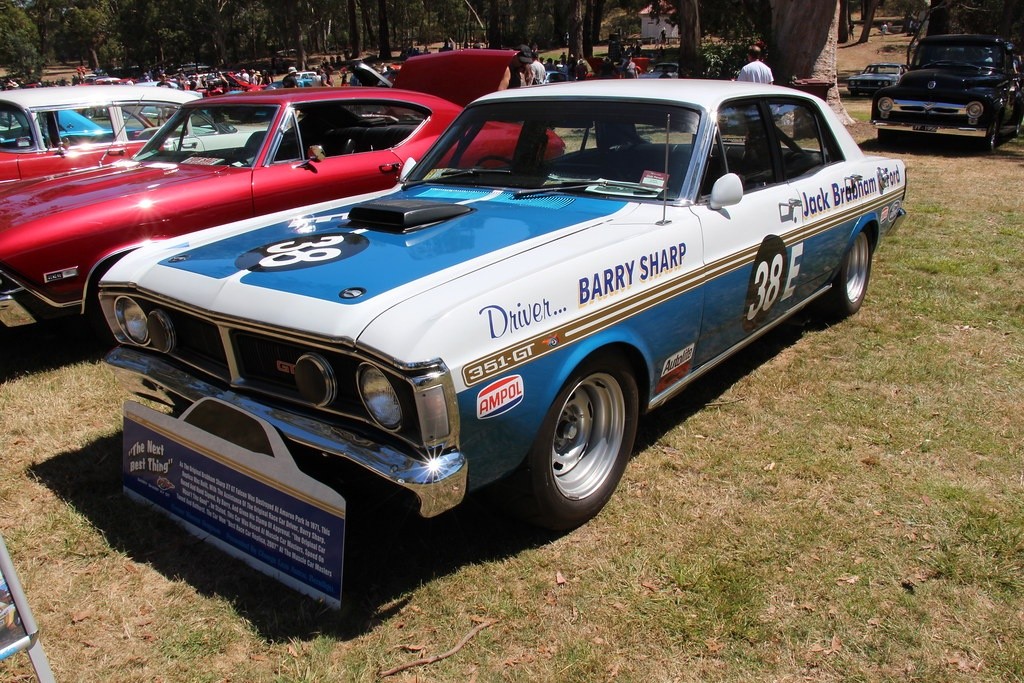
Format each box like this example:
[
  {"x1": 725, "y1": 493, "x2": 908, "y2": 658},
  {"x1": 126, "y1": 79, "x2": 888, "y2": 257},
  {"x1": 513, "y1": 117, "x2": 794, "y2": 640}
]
[
  {"x1": 607, "y1": 144, "x2": 819, "y2": 189},
  {"x1": 295, "y1": 125, "x2": 419, "y2": 157}
]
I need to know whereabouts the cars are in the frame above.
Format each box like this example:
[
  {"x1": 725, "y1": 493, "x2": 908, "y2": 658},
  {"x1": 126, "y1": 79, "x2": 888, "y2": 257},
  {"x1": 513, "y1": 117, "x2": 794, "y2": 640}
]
[
  {"x1": 544, "y1": 72, "x2": 570, "y2": 82},
  {"x1": 74, "y1": 78, "x2": 137, "y2": 119},
  {"x1": 641, "y1": 62, "x2": 679, "y2": 79},
  {"x1": 1, "y1": 85, "x2": 271, "y2": 178},
  {"x1": 868, "y1": 34, "x2": 1023, "y2": 152},
  {"x1": 178, "y1": 63, "x2": 210, "y2": 73},
  {"x1": 0, "y1": 108, "x2": 144, "y2": 143},
  {"x1": 96, "y1": 77, "x2": 910, "y2": 518},
  {"x1": 295, "y1": 71, "x2": 319, "y2": 80},
  {"x1": 215, "y1": 79, "x2": 322, "y2": 122},
  {"x1": 1, "y1": 49, "x2": 565, "y2": 317},
  {"x1": 102, "y1": 81, "x2": 208, "y2": 120},
  {"x1": 848, "y1": 62, "x2": 909, "y2": 96}
]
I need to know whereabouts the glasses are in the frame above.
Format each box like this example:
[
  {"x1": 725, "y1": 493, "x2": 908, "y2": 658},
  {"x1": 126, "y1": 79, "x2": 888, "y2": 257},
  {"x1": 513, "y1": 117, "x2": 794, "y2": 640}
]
[{"x1": 162, "y1": 76, "x2": 167, "y2": 78}]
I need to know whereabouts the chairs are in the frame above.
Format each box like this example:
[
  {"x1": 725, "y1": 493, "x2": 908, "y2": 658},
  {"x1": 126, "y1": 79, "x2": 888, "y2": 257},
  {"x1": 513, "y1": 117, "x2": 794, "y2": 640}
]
[{"x1": 226, "y1": 131, "x2": 267, "y2": 166}]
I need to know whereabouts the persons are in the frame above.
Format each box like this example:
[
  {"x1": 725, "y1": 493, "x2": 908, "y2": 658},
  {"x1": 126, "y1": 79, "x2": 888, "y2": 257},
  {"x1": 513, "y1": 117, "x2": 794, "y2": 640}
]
[
  {"x1": 314, "y1": 54, "x2": 395, "y2": 88},
  {"x1": 848, "y1": 22, "x2": 855, "y2": 40},
  {"x1": 522, "y1": 27, "x2": 675, "y2": 85},
  {"x1": 880, "y1": 22, "x2": 888, "y2": 40},
  {"x1": 498, "y1": 44, "x2": 532, "y2": 89},
  {"x1": 737, "y1": 43, "x2": 774, "y2": 85},
  {"x1": 399, "y1": 46, "x2": 432, "y2": 57},
  {"x1": 0, "y1": 57, "x2": 270, "y2": 99},
  {"x1": 282, "y1": 66, "x2": 299, "y2": 88}
]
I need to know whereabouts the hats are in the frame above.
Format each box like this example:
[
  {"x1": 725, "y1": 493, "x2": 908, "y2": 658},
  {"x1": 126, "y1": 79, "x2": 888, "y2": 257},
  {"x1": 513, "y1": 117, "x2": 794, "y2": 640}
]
[
  {"x1": 254, "y1": 71, "x2": 261, "y2": 75},
  {"x1": 328, "y1": 66, "x2": 335, "y2": 71},
  {"x1": 578, "y1": 59, "x2": 586, "y2": 65},
  {"x1": 288, "y1": 67, "x2": 297, "y2": 74},
  {"x1": 381, "y1": 63, "x2": 384, "y2": 65},
  {"x1": 241, "y1": 69, "x2": 245, "y2": 72},
  {"x1": 514, "y1": 44, "x2": 532, "y2": 63},
  {"x1": 249, "y1": 69, "x2": 255, "y2": 73},
  {"x1": 261, "y1": 70, "x2": 267, "y2": 75},
  {"x1": 532, "y1": 52, "x2": 538, "y2": 58}
]
